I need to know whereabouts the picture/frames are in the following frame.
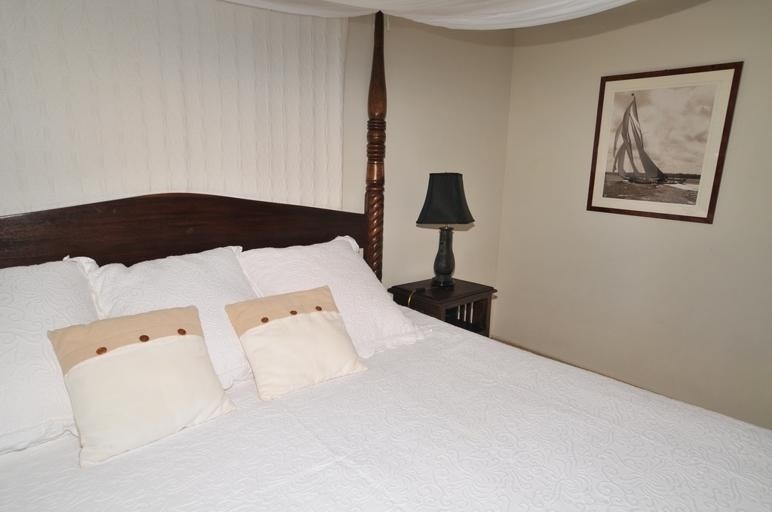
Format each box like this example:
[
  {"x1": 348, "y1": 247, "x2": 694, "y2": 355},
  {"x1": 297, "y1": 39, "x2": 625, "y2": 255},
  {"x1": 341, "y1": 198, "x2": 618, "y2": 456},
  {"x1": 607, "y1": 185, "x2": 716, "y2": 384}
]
[{"x1": 586, "y1": 62, "x2": 744, "y2": 224}]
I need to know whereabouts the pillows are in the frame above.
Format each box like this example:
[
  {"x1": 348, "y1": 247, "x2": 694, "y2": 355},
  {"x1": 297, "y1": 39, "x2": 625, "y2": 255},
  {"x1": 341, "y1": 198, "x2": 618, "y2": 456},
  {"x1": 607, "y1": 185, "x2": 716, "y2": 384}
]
[
  {"x1": 43, "y1": 303, "x2": 239, "y2": 470},
  {"x1": 242, "y1": 236, "x2": 413, "y2": 356},
  {"x1": 0, "y1": 255, "x2": 109, "y2": 457},
  {"x1": 223, "y1": 283, "x2": 370, "y2": 402},
  {"x1": 88, "y1": 241, "x2": 265, "y2": 394}
]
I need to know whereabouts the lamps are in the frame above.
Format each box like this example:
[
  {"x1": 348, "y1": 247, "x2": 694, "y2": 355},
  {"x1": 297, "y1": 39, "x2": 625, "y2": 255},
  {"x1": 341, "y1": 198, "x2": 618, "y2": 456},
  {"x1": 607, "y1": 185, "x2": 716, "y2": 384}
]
[{"x1": 415, "y1": 172, "x2": 475, "y2": 288}]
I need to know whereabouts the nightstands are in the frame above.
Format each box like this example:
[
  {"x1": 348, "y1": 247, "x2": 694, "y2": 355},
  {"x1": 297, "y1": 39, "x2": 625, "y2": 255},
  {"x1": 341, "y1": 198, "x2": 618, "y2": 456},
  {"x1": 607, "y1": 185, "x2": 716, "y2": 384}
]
[{"x1": 387, "y1": 277, "x2": 498, "y2": 338}]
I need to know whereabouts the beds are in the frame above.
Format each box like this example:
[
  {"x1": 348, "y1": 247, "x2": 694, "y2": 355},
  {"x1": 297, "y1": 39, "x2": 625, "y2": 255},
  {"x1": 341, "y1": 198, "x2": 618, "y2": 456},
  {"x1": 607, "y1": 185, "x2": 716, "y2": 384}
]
[{"x1": 1, "y1": 191, "x2": 770, "y2": 510}]
